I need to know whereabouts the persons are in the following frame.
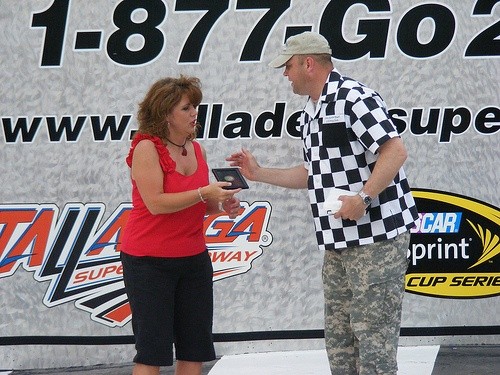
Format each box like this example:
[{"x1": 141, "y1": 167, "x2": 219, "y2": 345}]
[
  {"x1": 119, "y1": 76, "x2": 242, "y2": 375},
  {"x1": 225, "y1": 31, "x2": 420, "y2": 375}
]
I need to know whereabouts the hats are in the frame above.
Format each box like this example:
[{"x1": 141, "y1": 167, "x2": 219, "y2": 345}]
[{"x1": 268, "y1": 31, "x2": 332, "y2": 68}]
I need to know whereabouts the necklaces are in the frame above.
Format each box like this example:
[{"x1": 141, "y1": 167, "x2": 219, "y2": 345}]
[{"x1": 166, "y1": 137, "x2": 188, "y2": 156}]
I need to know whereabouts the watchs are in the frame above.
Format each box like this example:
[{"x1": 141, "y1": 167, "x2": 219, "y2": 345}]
[
  {"x1": 218, "y1": 202, "x2": 226, "y2": 213},
  {"x1": 357, "y1": 190, "x2": 374, "y2": 207}
]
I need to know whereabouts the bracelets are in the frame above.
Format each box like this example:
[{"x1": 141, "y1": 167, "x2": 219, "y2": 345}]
[{"x1": 197, "y1": 186, "x2": 207, "y2": 203}]
